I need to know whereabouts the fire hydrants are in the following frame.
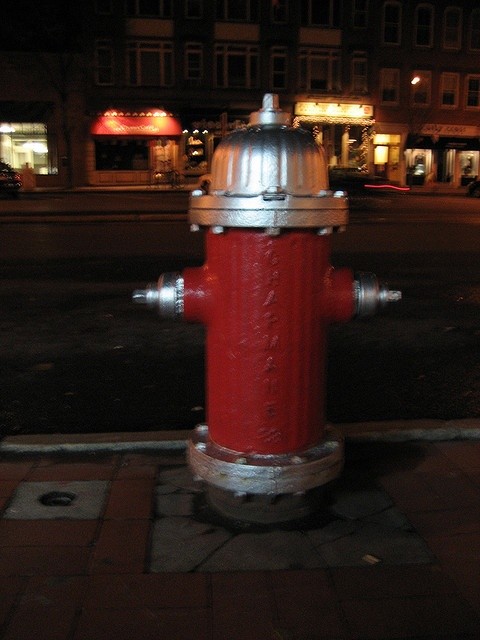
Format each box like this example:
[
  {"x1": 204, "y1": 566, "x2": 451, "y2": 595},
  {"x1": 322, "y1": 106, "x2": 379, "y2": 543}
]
[{"x1": 134, "y1": 93, "x2": 402, "y2": 525}]
